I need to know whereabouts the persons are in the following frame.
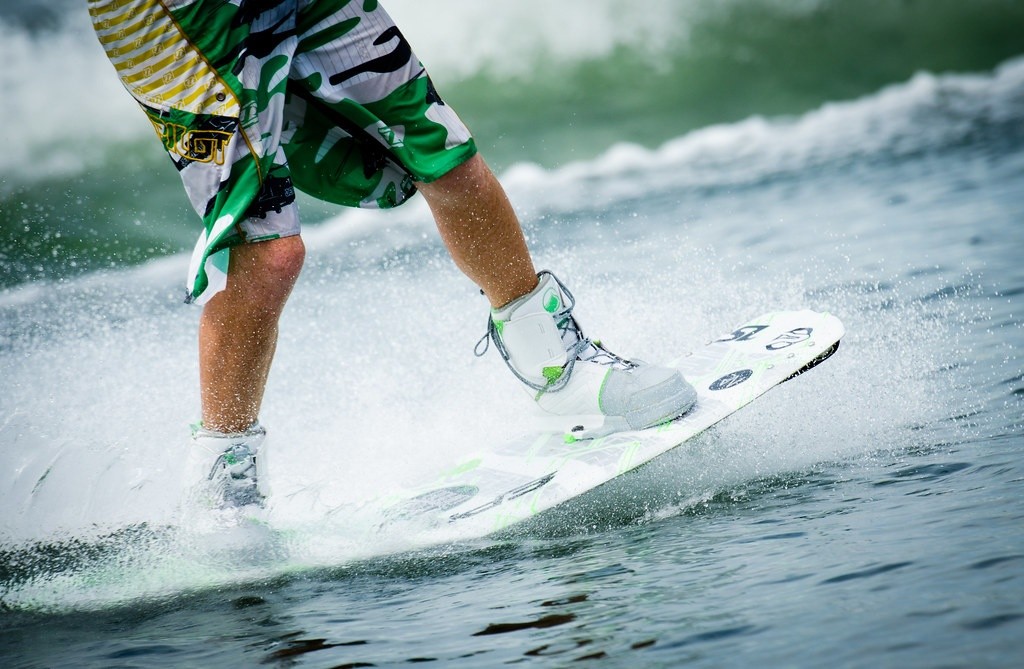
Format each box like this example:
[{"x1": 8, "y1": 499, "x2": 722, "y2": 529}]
[{"x1": 86, "y1": 0, "x2": 693, "y2": 557}]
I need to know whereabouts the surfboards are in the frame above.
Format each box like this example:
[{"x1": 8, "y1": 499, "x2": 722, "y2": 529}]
[{"x1": 4, "y1": 301, "x2": 848, "y2": 621}]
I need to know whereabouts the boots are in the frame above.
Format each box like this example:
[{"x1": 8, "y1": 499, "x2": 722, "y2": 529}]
[
  {"x1": 177, "y1": 420, "x2": 286, "y2": 568},
  {"x1": 489, "y1": 266, "x2": 696, "y2": 439}
]
